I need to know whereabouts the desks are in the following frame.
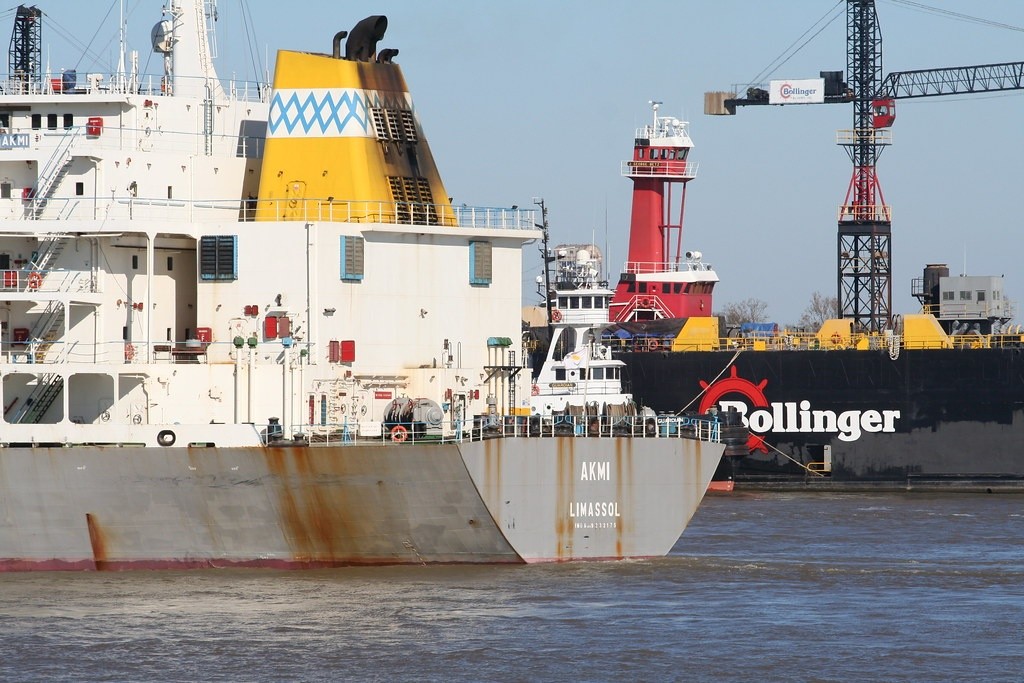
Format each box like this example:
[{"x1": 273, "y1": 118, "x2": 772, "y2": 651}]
[{"x1": 173, "y1": 347, "x2": 208, "y2": 364}]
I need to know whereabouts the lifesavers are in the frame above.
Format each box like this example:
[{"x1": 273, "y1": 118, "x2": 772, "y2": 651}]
[
  {"x1": 649, "y1": 340, "x2": 659, "y2": 351},
  {"x1": 552, "y1": 311, "x2": 562, "y2": 322},
  {"x1": 125, "y1": 343, "x2": 135, "y2": 359},
  {"x1": 27, "y1": 271, "x2": 42, "y2": 288},
  {"x1": 391, "y1": 426, "x2": 408, "y2": 442},
  {"x1": 531, "y1": 384, "x2": 539, "y2": 396}
]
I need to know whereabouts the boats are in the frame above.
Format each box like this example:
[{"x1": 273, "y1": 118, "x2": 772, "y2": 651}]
[
  {"x1": 0, "y1": 0, "x2": 729, "y2": 569},
  {"x1": 531, "y1": 0, "x2": 1024, "y2": 487}
]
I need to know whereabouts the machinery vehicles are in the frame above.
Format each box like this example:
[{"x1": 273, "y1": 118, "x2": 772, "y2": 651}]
[{"x1": 703, "y1": 0, "x2": 1024, "y2": 348}]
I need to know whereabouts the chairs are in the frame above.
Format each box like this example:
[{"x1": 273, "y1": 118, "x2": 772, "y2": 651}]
[{"x1": 153, "y1": 345, "x2": 171, "y2": 364}]
